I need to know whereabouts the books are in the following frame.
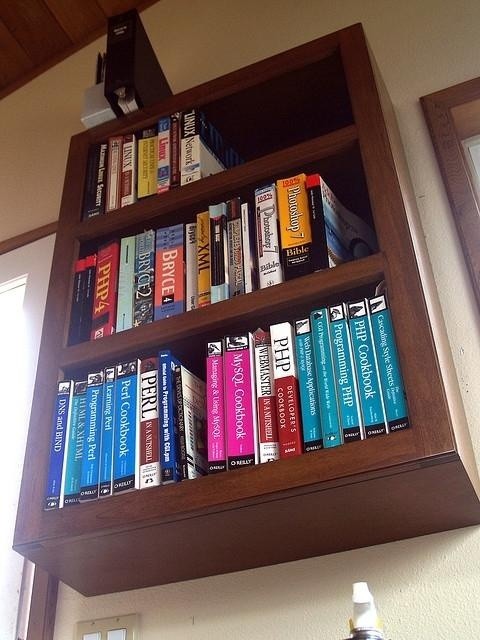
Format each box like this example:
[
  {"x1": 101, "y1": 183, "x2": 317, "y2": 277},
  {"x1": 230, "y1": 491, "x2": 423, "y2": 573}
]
[
  {"x1": 86, "y1": 104, "x2": 229, "y2": 217},
  {"x1": 37, "y1": 279, "x2": 406, "y2": 510},
  {"x1": 71, "y1": 172, "x2": 380, "y2": 339}
]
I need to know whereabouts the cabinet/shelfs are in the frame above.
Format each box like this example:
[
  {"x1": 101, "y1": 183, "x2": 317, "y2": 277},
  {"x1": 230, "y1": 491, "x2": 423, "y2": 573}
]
[{"x1": 12, "y1": 21, "x2": 480, "y2": 597}]
